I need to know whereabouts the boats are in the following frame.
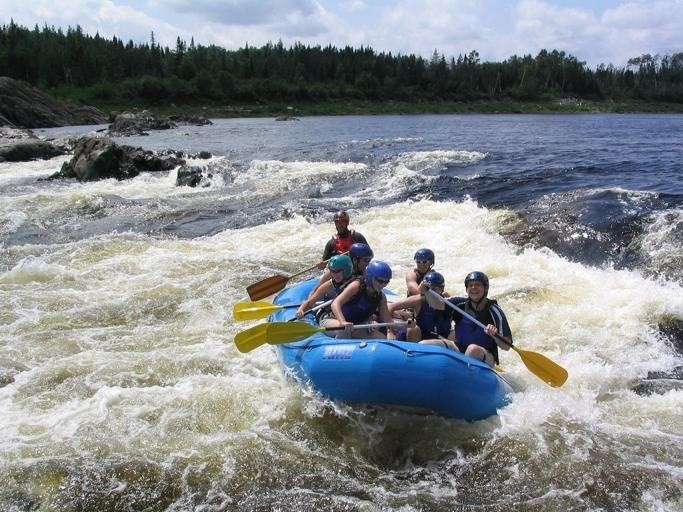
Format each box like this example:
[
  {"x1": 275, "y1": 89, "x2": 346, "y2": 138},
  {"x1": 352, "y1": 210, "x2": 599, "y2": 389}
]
[{"x1": 254, "y1": 277, "x2": 525, "y2": 424}]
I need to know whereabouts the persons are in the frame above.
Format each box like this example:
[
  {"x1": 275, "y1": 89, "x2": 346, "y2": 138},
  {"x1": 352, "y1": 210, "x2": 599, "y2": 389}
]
[
  {"x1": 401, "y1": 248, "x2": 450, "y2": 318},
  {"x1": 315, "y1": 210, "x2": 368, "y2": 270},
  {"x1": 306, "y1": 242, "x2": 375, "y2": 298},
  {"x1": 317, "y1": 259, "x2": 396, "y2": 340},
  {"x1": 294, "y1": 253, "x2": 354, "y2": 323},
  {"x1": 414, "y1": 270, "x2": 513, "y2": 371},
  {"x1": 386, "y1": 272, "x2": 470, "y2": 343}
]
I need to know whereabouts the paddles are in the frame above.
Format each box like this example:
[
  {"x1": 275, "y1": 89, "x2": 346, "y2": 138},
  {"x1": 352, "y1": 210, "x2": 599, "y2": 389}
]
[
  {"x1": 233, "y1": 301, "x2": 326, "y2": 320},
  {"x1": 247, "y1": 250, "x2": 349, "y2": 302},
  {"x1": 425, "y1": 284, "x2": 568, "y2": 388},
  {"x1": 266, "y1": 319, "x2": 411, "y2": 345},
  {"x1": 234, "y1": 299, "x2": 335, "y2": 353}
]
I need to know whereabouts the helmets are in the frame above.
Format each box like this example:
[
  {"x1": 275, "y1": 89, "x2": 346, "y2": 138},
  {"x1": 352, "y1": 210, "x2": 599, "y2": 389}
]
[
  {"x1": 328, "y1": 255, "x2": 353, "y2": 279},
  {"x1": 423, "y1": 272, "x2": 445, "y2": 288},
  {"x1": 415, "y1": 249, "x2": 434, "y2": 265},
  {"x1": 465, "y1": 271, "x2": 489, "y2": 289},
  {"x1": 349, "y1": 243, "x2": 374, "y2": 258},
  {"x1": 366, "y1": 260, "x2": 391, "y2": 289},
  {"x1": 332, "y1": 211, "x2": 349, "y2": 225}
]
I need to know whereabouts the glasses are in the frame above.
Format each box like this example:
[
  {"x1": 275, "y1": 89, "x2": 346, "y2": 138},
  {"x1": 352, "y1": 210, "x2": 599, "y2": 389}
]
[
  {"x1": 331, "y1": 269, "x2": 343, "y2": 273},
  {"x1": 378, "y1": 280, "x2": 389, "y2": 284},
  {"x1": 416, "y1": 260, "x2": 427, "y2": 264}
]
